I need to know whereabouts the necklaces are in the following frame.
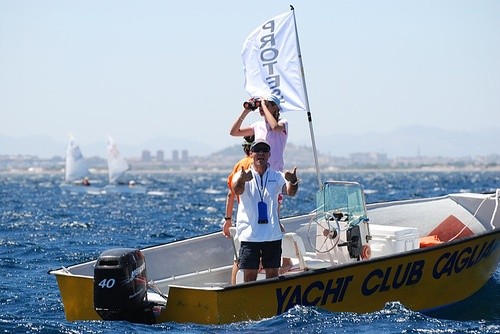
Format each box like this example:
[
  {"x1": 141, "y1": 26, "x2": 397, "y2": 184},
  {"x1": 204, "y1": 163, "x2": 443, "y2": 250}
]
[{"x1": 291, "y1": 178, "x2": 300, "y2": 186}]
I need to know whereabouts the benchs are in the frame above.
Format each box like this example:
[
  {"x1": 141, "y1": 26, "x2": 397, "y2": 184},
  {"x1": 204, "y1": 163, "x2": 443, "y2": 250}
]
[{"x1": 227, "y1": 225, "x2": 331, "y2": 284}]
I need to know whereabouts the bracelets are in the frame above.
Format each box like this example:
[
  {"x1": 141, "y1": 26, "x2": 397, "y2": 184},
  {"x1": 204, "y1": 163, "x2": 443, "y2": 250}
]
[
  {"x1": 224, "y1": 217, "x2": 231, "y2": 220},
  {"x1": 231, "y1": 138, "x2": 300, "y2": 283},
  {"x1": 239, "y1": 117, "x2": 244, "y2": 121}
]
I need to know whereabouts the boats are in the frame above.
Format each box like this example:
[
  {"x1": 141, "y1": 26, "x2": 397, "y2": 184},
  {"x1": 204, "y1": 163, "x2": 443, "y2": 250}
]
[{"x1": 47, "y1": 186, "x2": 500, "y2": 326}]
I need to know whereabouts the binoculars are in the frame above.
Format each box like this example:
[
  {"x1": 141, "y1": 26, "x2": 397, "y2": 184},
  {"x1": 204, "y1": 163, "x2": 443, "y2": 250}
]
[{"x1": 244, "y1": 101, "x2": 261, "y2": 110}]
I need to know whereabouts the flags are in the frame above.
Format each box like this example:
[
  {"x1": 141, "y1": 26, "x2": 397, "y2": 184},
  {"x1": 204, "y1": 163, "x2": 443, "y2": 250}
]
[{"x1": 241, "y1": 10, "x2": 307, "y2": 112}]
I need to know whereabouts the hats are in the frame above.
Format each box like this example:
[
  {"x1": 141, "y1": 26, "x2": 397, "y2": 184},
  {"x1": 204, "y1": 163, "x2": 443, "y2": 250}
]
[
  {"x1": 242, "y1": 135, "x2": 254, "y2": 145},
  {"x1": 264, "y1": 94, "x2": 283, "y2": 110},
  {"x1": 250, "y1": 139, "x2": 271, "y2": 150}
]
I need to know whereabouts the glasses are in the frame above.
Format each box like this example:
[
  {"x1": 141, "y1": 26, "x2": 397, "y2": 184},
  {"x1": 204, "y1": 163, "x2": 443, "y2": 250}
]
[{"x1": 252, "y1": 148, "x2": 269, "y2": 152}]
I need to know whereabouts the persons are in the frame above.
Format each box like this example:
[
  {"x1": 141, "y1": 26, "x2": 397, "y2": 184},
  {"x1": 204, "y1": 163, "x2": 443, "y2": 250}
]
[
  {"x1": 230, "y1": 94, "x2": 293, "y2": 275},
  {"x1": 223, "y1": 136, "x2": 255, "y2": 286},
  {"x1": 83, "y1": 176, "x2": 90, "y2": 187}
]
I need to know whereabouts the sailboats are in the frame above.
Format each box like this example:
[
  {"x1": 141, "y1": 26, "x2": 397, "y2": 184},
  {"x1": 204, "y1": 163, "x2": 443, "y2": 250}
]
[
  {"x1": 101, "y1": 136, "x2": 148, "y2": 194},
  {"x1": 57, "y1": 134, "x2": 103, "y2": 193}
]
